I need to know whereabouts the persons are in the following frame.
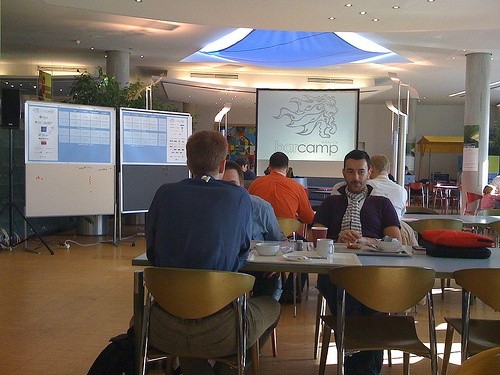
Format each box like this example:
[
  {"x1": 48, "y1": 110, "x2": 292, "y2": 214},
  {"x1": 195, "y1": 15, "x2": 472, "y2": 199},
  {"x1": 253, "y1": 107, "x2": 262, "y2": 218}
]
[
  {"x1": 145, "y1": 130, "x2": 282, "y2": 375},
  {"x1": 365, "y1": 153, "x2": 407, "y2": 225},
  {"x1": 312, "y1": 150, "x2": 402, "y2": 375},
  {"x1": 247, "y1": 151, "x2": 315, "y2": 302},
  {"x1": 481, "y1": 185, "x2": 500, "y2": 210},
  {"x1": 222, "y1": 160, "x2": 288, "y2": 301},
  {"x1": 404, "y1": 166, "x2": 415, "y2": 185},
  {"x1": 234, "y1": 156, "x2": 294, "y2": 180}
]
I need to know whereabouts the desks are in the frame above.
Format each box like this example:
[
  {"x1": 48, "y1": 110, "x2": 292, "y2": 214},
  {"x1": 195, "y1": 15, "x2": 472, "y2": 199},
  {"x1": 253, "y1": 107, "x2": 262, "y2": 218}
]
[
  {"x1": 434, "y1": 186, "x2": 461, "y2": 215},
  {"x1": 132, "y1": 239, "x2": 499, "y2": 364}
]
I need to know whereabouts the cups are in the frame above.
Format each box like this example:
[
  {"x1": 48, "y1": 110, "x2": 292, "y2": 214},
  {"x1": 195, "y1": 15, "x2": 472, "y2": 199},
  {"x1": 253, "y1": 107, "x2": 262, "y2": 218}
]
[
  {"x1": 311, "y1": 227, "x2": 328, "y2": 248},
  {"x1": 317, "y1": 238, "x2": 334, "y2": 258}
]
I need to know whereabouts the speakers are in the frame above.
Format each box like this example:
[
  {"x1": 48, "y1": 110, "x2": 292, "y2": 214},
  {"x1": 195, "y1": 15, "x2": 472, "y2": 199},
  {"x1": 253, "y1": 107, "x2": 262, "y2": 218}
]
[{"x1": 0, "y1": 88, "x2": 20, "y2": 129}]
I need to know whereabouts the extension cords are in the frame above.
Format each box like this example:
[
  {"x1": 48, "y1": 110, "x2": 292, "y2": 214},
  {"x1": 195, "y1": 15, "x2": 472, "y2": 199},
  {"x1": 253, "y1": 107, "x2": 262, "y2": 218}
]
[{"x1": 57, "y1": 244, "x2": 70, "y2": 249}]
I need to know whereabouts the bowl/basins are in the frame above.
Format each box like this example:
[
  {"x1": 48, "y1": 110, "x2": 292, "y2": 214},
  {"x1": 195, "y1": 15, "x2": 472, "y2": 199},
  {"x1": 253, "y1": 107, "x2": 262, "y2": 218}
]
[
  {"x1": 378, "y1": 241, "x2": 400, "y2": 251},
  {"x1": 256, "y1": 243, "x2": 279, "y2": 256}
]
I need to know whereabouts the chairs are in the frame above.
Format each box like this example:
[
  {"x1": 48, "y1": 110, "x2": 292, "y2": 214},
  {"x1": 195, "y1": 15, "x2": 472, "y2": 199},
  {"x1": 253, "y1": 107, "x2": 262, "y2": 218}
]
[
  {"x1": 441, "y1": 268, "x2": 500, "y2": 375},
  {"x1": 407, "y1": 181, "x2": 500, "y2": 237},
  {"x1": 406, "y1": 218, "x2": 463, "y2": 300},
  {"x1": 318, "y1": 265, "x2": 437, "y2": 375},
  {"x1": 276, "y1": 218, "x2": 310, "y2": 317},
  {"x1": 138, "y1": 265, "x2": 261, "y2": 375}
]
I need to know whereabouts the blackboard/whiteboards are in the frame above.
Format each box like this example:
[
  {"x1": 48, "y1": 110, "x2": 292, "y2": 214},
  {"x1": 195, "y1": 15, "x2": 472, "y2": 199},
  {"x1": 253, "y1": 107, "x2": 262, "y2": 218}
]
[
  {"x1": 25, "y1": 100, "x2": 116, "y2": 217},
  {"x1": 119, "y1": 106, "x2": 192, "y2": 214}
]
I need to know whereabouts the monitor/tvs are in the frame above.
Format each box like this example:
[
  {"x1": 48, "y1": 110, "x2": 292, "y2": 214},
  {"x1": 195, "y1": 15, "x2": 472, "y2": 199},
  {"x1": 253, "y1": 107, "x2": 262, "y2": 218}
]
[{"x1": 405, "y1": 175, "x2": 415, "y2": 184}]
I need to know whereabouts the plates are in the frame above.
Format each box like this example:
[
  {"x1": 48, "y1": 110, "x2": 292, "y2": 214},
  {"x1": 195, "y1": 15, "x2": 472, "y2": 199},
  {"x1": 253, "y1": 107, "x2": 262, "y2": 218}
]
[{"x1": 282, "y1": 253, "x2": 309, "y2": 261}]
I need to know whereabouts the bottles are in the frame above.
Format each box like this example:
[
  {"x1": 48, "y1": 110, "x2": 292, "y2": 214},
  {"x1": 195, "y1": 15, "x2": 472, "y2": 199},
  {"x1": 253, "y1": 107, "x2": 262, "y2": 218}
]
[{"x1": 296, "y1": 240, "x2": 303, "y2": 251}]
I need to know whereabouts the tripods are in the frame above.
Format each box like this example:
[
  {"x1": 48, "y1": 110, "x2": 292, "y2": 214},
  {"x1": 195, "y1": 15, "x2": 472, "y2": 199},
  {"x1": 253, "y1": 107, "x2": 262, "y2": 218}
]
[{"x1": 0, "y1": 128, "x2": 55, "y2": 255}]
